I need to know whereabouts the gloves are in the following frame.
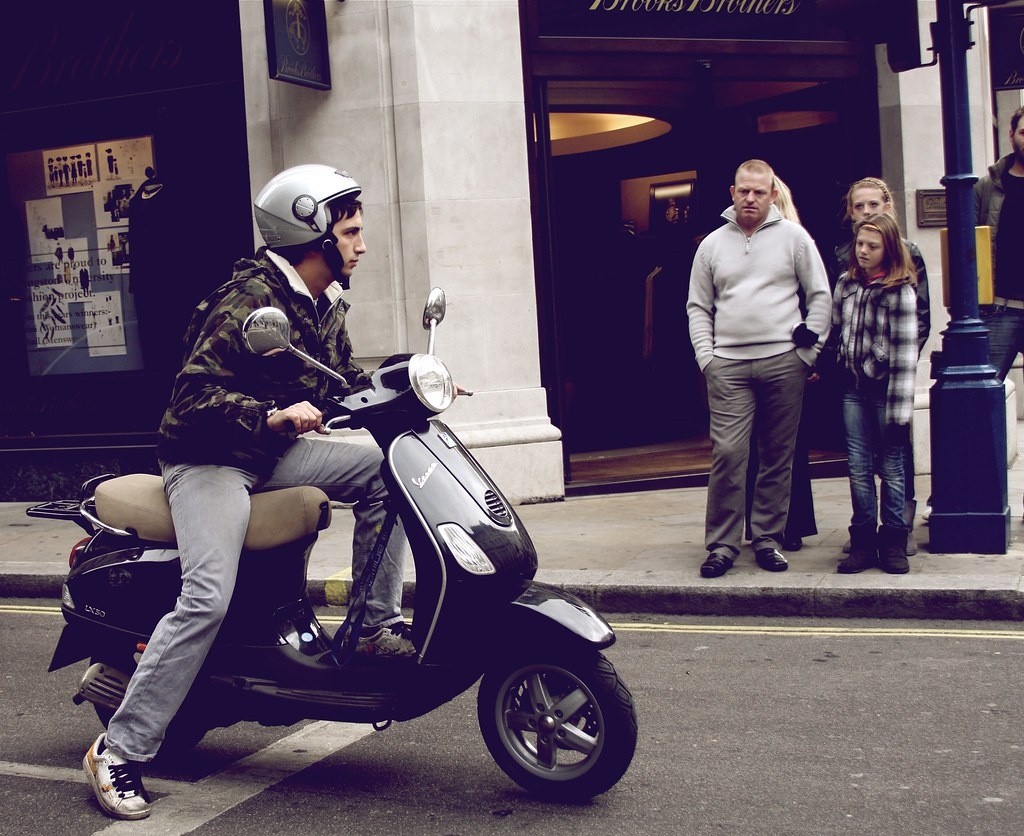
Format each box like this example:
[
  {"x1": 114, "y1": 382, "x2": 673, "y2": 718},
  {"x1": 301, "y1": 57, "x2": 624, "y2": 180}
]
[{"x1": 792, "y1": 322, "x2": 819, "y2": 348}]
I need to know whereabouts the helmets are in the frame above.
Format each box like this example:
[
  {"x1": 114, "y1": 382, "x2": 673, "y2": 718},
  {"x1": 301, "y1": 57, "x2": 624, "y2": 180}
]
[{"x1": 254, "y1": 164, "x2": 361, "y2": 249}]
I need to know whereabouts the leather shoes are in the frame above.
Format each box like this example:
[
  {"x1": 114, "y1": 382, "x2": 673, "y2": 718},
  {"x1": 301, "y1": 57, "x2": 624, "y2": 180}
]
[
  {"x1": 700, "y1": 552, "x2": 733, "y2": 577},
  {"x1": 755, "y1": 548, "x2": 788, "y2": 571}
]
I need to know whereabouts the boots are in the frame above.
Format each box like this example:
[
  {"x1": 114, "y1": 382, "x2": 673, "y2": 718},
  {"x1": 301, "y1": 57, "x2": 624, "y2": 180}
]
[
  {"x1": 842, "y1": 540, "x2": 851, "y2": 554},
  {"x1": 879, "y1": 525, "x2": 910, "y2": 574},
  {"x1": 837, "y1": 524, "x2": 877, "y2": 574},
  {"x1": 902, "y1": 500, "x2": 917, "y2": 556}
]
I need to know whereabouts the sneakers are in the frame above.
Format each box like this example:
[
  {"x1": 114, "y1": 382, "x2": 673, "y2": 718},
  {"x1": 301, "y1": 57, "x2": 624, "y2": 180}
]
[
  {"x1": 346, "y1": 621, "x2": 416, "y2": 660},
  {"x1": 83, "y1": 733, "x2": 151, "y2": 820}
]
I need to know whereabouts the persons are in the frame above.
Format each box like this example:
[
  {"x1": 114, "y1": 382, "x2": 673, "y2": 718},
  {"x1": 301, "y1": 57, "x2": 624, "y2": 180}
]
[
  {"x1": 83, "y1": 164, "x2": 465, "y2": 820},
  {"x1": 687, "y1": 159, "x2": 832, "y2": 577},
  {"x1": 791, "y1": 177, "x2": 930, "y2": 575},
  {"x1": 973, "y1": 104, "x2": 1024, "y2": 381}
]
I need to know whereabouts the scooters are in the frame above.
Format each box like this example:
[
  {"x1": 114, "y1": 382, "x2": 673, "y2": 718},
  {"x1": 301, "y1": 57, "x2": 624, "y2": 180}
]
[{"x1": 24, "y1": 287, "x2": 639, "y2": 802}]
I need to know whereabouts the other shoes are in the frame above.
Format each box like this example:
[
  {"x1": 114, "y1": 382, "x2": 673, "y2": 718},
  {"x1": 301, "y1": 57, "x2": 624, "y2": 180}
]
[
  {"x1": 782, "y1": 537, "x2": 802, "y2": 551},
  {"x1": 922, "y1": 507, "x2": 932, "y2": 520}
]
